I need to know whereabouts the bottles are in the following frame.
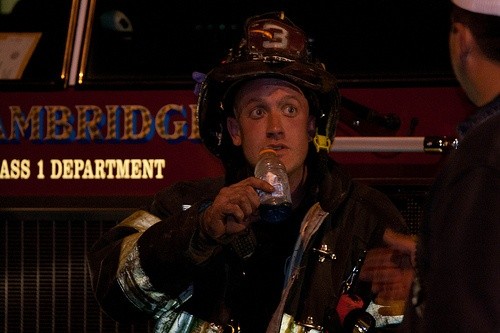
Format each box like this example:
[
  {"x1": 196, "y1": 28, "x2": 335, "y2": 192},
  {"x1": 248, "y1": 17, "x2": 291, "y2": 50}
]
[{"x1": 254, "y1": 146, "x2": 294, "y2": 221}]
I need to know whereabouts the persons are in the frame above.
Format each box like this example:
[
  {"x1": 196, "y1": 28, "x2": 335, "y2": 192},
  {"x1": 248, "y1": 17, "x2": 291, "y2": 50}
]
[
  {"x1": 361, "y1": 1, "x2": 500, "y2": 332},
  {"x1": 88, "y1": 10, "x2": 415, "y2": 333}
]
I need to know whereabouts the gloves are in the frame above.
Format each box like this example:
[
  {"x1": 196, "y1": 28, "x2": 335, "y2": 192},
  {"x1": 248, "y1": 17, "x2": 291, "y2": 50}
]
[{"x1": 201, "y1": 176, "x2": 274, "y2": 240}]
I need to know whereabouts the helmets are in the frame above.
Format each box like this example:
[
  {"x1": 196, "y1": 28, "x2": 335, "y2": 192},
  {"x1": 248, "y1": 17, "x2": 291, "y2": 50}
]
[{"x1": 195, "y1": 13, "x2": 339, "y2": 163}]
